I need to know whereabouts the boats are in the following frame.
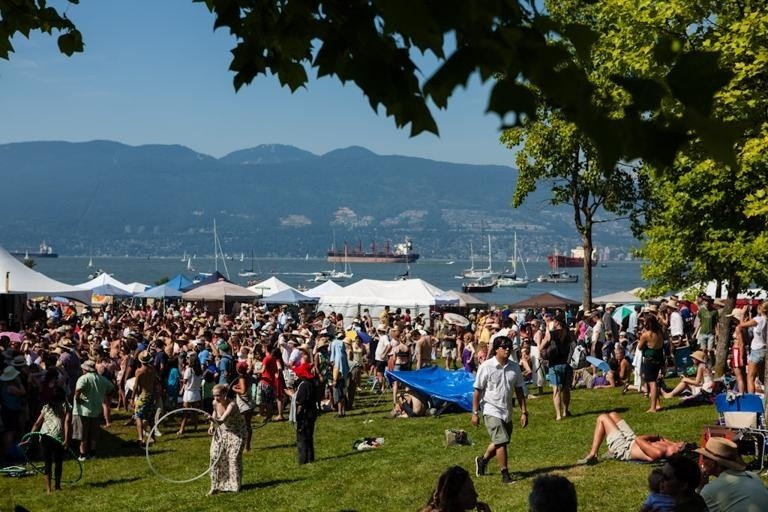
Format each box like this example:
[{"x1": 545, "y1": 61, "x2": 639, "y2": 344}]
[
  {"x1": 547, "y1": 254, "x2": 598, "y2": 268},
  {"x1": 326, "y1": 239, "x2": 419, "y2": 263},
  {"x1": 536, "y1": 275, "x2": 580, "y2": 283},
  {"x1": 88, "y1": 273, "x2": 114, "y2": 280},
  {"x1": 9, "y1": 250, "x2": 58, "y2": 258}
]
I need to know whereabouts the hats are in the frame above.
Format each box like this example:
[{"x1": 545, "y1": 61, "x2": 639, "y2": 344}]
[
  {"x1": 295, "y1": 363, "x2": 313, "y2": 379},
  {"x1": 377, "y1": 324, "x2": 387, "y2": 331},
  {"x1": 725, "y1": 308, "x2": 744, "y2": 321},
  {"x1": 584, "y1": 303, "x2": 616, "y2": 318},
  {"x1": 690, "y1": 351, "x2": 706, "y2": 363},
  {"x1": 692, "y1": 437, "x2": 746, "y2": 471},
  {"x1": 235, "y1": 303, "x2": 264, "y2": 320},
  {"x1": 42, "y1": 302, "x2": 90, "y2": 314},
  {"x1": 235, "y1": 362, "x2": 248, "y2": 368},
  {"x1": 285, "y1": 316, "x2": 360, "y2": 352},
  {"x1": 619, "y1": 332, "x2": 626, "y2": 336},
  {"x1": 635, "y1": 292, "x2": 725, "y2": 312},
  {"x1": 138, "y1": 304, "x2": 229, "y2": 363},
  {"x1": 0, "y1": 327, "x2": 99, "y2": 381},
  {"x1": 509, "y1": 314, "x2": 517, "y2": 321}
]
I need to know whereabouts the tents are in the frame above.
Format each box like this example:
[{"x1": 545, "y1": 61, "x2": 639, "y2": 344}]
[{"x1": 1, "y1": 247, "x2": 486, "y2": 336}]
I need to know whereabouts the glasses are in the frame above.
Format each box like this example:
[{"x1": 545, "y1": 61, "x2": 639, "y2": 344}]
[{"x1": 499, "y1": 345, "x2": 513, "y2": 349}]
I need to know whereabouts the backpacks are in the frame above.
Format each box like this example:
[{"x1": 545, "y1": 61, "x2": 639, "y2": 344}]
[
  {"x1": 222, "y1": 356, "x2": 239, "y2": 384},
  {"x1": 237, "y1": 376, "x2": 272, "y2": 407}
]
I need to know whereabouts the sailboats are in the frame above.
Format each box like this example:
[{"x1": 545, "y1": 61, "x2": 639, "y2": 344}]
[
  {"x1": 314, "y1": 244, "x2": 354, "y2": 282},
  {"x1": 180, "y1": 250, "x2": 195, "y2": 271},
  {"x1": 192, "y1": 217, "x2": 231, "y2": 285},
  {"x1": 237, "y1": 248, "x2": 257, "y2": 278},
  {"x1": 462, "y1": 231, "x2": 530, "y2": 293}
]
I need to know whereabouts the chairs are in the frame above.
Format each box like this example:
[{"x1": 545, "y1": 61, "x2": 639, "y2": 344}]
[{"x1": 714, "y1": 393, "x2": 768, "y2": 455}]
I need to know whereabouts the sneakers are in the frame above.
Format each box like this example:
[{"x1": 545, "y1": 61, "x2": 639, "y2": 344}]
[
  {"x1": 124, "y1": 421, "x2": 161, "y2": 446},
  {"x1": 578, "y1": 455, "x2": 597, "y2": 464},
  {"x1": 475, "y1": 456, "x2": 486, "y2": 477},
  {"x1": 501, "y1": 476, "x2": 516, "y2": 483}
]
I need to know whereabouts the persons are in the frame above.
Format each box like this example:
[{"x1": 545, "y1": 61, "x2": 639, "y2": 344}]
[
  {"x1": 19, "y1": 384, "x2": 71, "y2": 496},
  {"x1": 206, "y1": 386, "x2": 246, "y2": 495},
  {"x1": 641, "y1": 457, "x2": 708, "y2": 511},
  {"x1": 470, "y1": 336, "x2": 529, "y2": 484},
  {"x1": 691, "y1": 437, "x2": 768, "y2": 512},
  {"x1": 530, "y1": 475, "x2": 577, "y2": 511},
  {"x1": 0, "y1": 292, "x2": 767, "y2": 466},
  {"x1": 417, "y1": 465, "x2": 490, "y2": 511}
]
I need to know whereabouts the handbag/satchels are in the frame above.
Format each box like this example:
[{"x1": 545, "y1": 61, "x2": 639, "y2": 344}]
[{"x1": 540, "y1": 332, "x2": 558, "y2": 360}]
